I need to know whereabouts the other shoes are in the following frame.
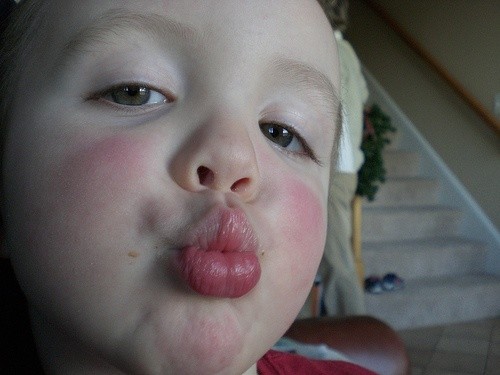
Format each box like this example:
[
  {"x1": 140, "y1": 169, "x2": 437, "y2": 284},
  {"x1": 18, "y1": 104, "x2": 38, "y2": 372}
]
[
  {"x1": 365, "y1": 278, "x2": 382, "y2": 293},
  {"x1": 383, "y1": 274, "x2": 403, "y2": 289}
]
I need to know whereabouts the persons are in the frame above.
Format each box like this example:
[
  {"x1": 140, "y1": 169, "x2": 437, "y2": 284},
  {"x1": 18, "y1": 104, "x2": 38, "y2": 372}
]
[
  {"x1": 316, "y1": 0, "x2": 369, "y2": 316},
  {"x1": 0, "y1": 0, "x2": 382, "y2": 375}
]
